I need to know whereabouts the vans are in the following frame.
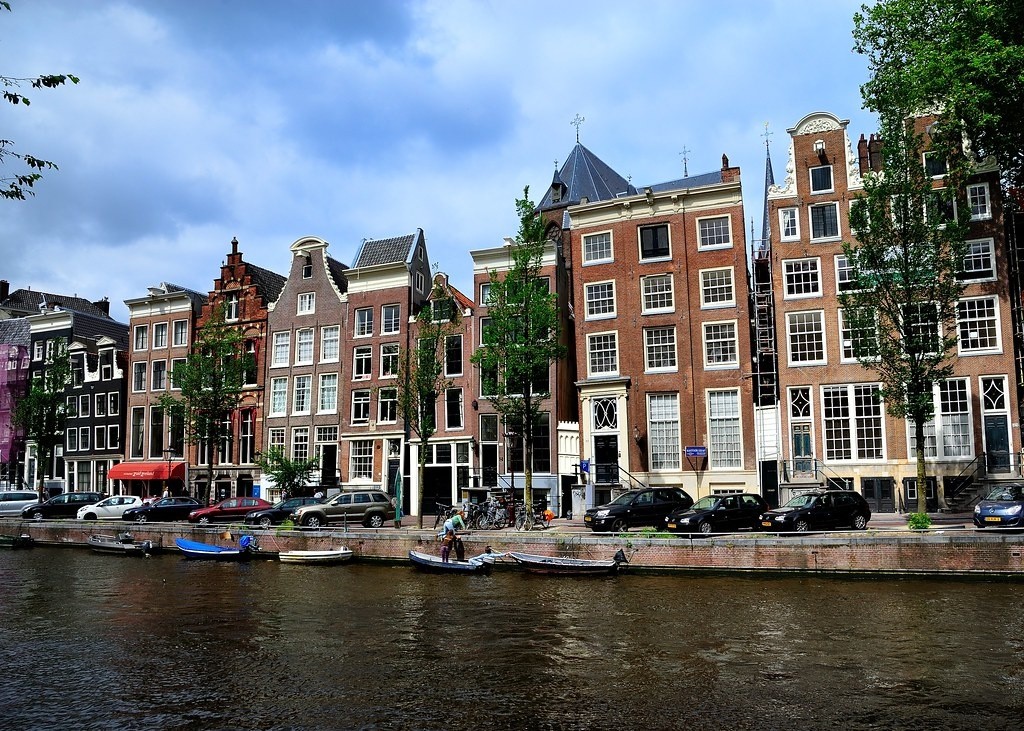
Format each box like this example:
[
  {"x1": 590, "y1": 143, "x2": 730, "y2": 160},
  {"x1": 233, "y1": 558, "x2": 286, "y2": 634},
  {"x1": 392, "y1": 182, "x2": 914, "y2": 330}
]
[{"x1": 0, "y1": 491, "x2": 50, "y2": 518}]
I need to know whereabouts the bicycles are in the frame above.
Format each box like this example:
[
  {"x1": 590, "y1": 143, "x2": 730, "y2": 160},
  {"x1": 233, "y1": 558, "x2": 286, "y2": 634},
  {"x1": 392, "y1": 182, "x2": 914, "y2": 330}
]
[{"x1": 432, "y1": 499, "x2": 554, "y2": 533}]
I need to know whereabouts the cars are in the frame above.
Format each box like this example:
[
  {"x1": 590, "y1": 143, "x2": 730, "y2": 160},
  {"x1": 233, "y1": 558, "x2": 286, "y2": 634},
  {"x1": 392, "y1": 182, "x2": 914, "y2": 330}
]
[
  {"x1": 76, "y1": 495, "x2": 144, "y2": 524},
  {"x1": 188, "y1": 497, "x2": 273, "y2": 528},
  {"x1": 122, "y1": 496, "x2": 214, "y2": 525},
  {"x1": 759, "y1": 490, "x2": 872, "y2": 536},
  {"x1": 972, "y1": 482, "x2": 1024, "y2": 532},
  {"x1": 21, "y1": 492, "x2": 102, "y2": 523},
  {"x1": 664, "y1": 493, "x2": 769, "y2": 538},
  {"x1": 243, "y1": 496, "x2": 326, "y2": 530}
]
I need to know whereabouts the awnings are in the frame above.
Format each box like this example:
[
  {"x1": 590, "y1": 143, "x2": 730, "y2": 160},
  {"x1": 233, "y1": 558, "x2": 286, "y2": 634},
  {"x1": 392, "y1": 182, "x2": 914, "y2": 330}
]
[{"x1": 107, "y1": 462, "x2": 184, "y2": 482}]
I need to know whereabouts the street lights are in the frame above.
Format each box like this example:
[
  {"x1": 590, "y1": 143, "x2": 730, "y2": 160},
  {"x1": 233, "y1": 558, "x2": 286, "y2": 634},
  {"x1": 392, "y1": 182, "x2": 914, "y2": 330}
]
[{"x1": 163, "y1": 448, "x2": 175, "y2": 497}]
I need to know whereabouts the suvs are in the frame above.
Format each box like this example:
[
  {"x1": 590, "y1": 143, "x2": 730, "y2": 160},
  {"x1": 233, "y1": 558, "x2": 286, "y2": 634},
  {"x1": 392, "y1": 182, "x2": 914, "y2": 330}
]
[
  {"x1": 584, "y1": 486, "x2": 695, "y2": 536},
  {"x1": 292, "y1": 489, "x2": 405, "y2": 531}
]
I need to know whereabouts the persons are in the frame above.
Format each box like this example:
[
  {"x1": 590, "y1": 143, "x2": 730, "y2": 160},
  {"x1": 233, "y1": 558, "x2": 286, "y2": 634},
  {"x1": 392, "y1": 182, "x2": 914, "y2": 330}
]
[
  {"x1": 440, "y1": 531, "x2": 453, "y2": 562},
  {"x1": 163, "y1": 487, "x2": 172, "y2": 498},
  {"x1": 433, "y1": 511, "x2": 467, "y2": 541}
]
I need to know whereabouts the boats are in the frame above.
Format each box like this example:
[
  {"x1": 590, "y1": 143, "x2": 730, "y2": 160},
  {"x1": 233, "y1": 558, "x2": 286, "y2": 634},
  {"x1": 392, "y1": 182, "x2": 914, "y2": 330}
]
[
  {"x1": 278, "y1": 545, "x2": 353, "y2": 564},
  {"x1": 0, "y1": 534, "x2": 35, "y2": 549},
  {"x1": 175, "y1": 535, "x2": 263, "y2": 558},
  {"x1": 408, "y1": 550, "x2": 491, "y2": 570},
  {"x1": 87, "y1": 532, "x2": 153, "y2": 556},
  {"x1": 508, "y1": 548, "x2": 629, "y2": 575}
]
